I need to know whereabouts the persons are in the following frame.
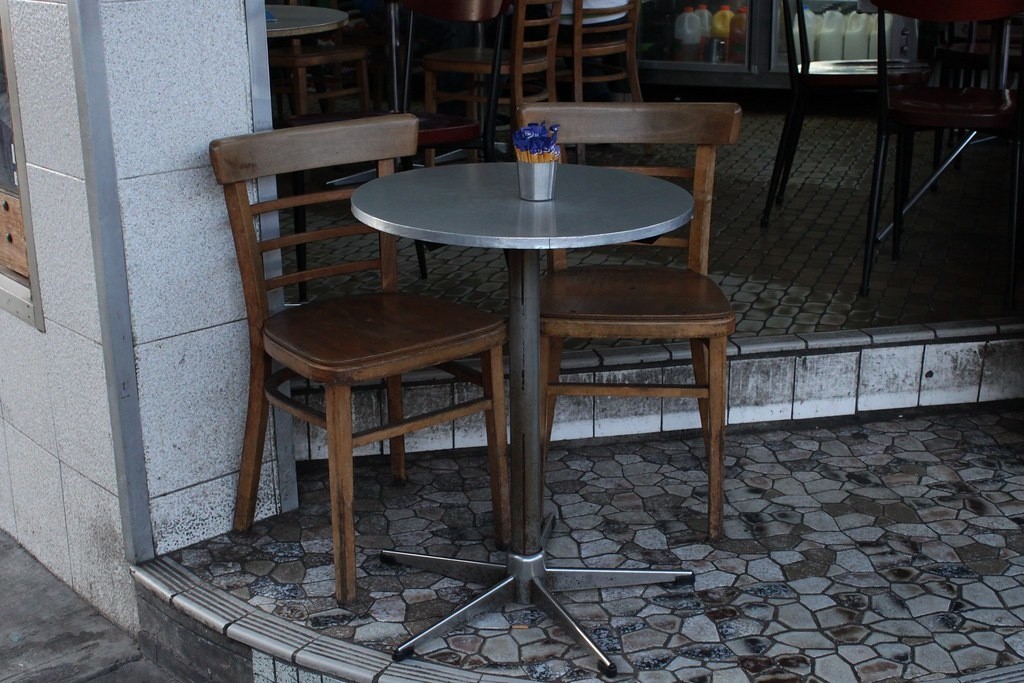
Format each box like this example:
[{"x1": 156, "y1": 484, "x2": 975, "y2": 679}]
[{"x1": 545, "y1": 0, "x2": 633, "y2": 102}]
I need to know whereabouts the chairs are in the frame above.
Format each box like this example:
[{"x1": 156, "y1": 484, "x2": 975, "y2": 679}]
[
  {"x1": 209, "y1": 112, "x2": 513, "y2": 607},
  {"x1": 519, "y1": 99, "x2": 745, "y2": 541},
  {"x1": 262, "y1": 0, "x2": 1023, "y2": 309}
]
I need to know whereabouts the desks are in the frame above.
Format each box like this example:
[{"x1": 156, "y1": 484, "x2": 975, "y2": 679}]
[
  {"x1": 353, "y1": 157, "x2": 695, "y2": 679},
  {"x1": 261, "y1": 6, "x2": 351, "y2": 38}
]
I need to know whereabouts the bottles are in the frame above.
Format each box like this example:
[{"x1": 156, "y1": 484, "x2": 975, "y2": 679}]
[
  {"x1": 730, "y1": 6, "x2": 748, "y2": 63},
  {"x1": 712, "y1": 6, "x2": 736, "y2": 63},
  {"x1": 694, "y1": 4, "x2": 713, "y2": 61},
  {"x1": 672, "y1": 7, "x2": 701, "y2": 62},
  {"x1": 792, "y1": 4, "x2": 819, "y2": 64},
  {"x1": 845, "y1": 11, "x2": 870, "y2": 59},
  {"x1": 818, "y1": 8, "x2": 844, "y2": 60},
  {"x1": 869, "y1": 13, "x2": 893, "y2": 60}
]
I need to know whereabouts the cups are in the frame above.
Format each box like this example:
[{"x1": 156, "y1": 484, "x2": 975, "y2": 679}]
[
  {"x1": 709, "y1": 37, "x2": 726, "y2": 63},
  {"x1": 516, "y1": 160, "x2": 558, "y2": 201}
]
